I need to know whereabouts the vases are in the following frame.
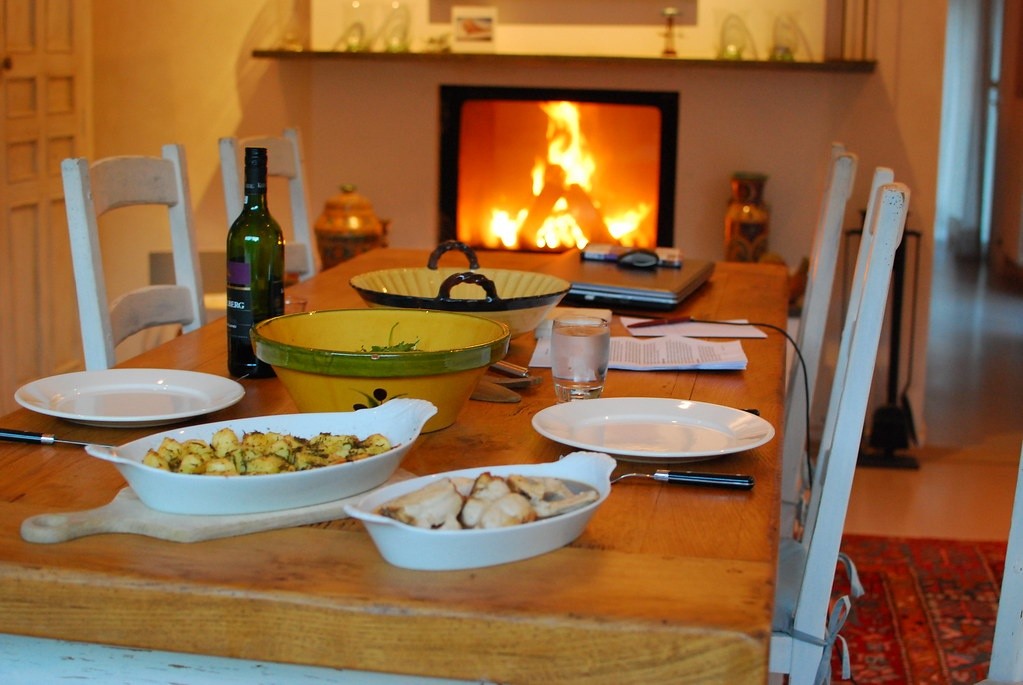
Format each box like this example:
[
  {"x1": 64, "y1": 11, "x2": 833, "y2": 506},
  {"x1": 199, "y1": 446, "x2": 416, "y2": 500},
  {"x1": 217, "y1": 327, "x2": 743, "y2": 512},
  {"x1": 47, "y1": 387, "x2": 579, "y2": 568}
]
[{"x1": 724, "y1": 172, "x2": 774, "y2": 263}]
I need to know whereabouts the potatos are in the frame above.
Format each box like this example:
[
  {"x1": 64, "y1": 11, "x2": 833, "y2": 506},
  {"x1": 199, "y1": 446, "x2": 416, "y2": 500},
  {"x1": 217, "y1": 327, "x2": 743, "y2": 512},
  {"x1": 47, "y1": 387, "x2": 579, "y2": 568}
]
[{"x1": 142, "y1": 428, "x2": 392, "y2": 475}]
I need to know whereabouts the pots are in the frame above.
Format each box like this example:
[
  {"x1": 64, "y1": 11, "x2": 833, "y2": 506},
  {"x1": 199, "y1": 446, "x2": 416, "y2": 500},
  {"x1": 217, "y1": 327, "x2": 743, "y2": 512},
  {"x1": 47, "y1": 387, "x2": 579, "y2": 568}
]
[{"x1": 347, "y1": 238, "x2": 574, "y2": 341}]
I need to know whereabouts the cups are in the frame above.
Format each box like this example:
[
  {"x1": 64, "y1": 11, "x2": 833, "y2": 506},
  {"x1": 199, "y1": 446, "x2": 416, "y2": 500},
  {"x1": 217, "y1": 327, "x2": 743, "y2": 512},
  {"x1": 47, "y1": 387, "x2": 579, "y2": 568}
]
[{"x1": 550, "y1": 313, "x2": 611, "y2": 405}]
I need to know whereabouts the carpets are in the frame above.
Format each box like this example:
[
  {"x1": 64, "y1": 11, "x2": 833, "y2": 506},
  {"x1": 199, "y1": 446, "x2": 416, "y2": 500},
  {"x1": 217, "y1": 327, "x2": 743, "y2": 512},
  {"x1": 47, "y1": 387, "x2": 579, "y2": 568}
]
[{"x1": 784, "y1": 529, "x2": 1007, "y2": 685}]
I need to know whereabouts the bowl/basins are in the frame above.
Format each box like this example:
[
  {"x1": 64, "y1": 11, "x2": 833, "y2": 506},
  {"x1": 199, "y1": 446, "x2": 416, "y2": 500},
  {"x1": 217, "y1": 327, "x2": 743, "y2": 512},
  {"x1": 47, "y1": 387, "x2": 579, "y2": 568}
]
[{"x1": 248, "y1": 305, "x2": 510, "y2": 437}]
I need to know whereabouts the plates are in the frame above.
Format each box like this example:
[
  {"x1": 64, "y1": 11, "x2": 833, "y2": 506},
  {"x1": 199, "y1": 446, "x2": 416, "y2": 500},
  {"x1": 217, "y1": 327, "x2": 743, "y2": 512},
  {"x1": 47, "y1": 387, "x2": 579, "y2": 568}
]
[
  {"x1": 531, "y1": 397, "x2": 776, "y2": 464},
  {"x1": 87, "y1": 398, "x2": 439, "y2": 517},
  {"x1": 341, "y1": 449, "x2": 617, "y2": 570},
  {"x1": 13, "y1": 367, "x2": 246, "y2": 427}
]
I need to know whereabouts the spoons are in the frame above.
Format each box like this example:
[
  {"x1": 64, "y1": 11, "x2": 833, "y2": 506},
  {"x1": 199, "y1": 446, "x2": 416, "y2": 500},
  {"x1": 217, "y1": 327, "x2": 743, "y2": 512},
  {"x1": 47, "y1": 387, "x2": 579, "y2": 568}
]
[{"x1": 470, "y1": 375, "x2": 543, "y2": 403}]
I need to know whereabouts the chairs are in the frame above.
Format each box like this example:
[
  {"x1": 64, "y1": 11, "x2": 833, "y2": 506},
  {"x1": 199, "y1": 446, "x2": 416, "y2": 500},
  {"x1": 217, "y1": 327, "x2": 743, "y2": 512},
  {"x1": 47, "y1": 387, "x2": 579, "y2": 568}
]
[
  {"x1": 61, "y1": 143, "x2": 208, "y2": 372},
  {"x1": 770, "y1": 141, "x2": 911, "y2": 685},
  {"x1": 218, "y1": 131, "x2": 320, "y2": 283}
]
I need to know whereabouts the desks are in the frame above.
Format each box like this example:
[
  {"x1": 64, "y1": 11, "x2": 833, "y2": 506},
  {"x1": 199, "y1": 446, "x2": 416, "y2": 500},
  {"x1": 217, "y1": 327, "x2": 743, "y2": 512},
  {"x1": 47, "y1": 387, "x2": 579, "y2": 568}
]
[{"x1": 0, "y1": 247, "x2": 791, "y2": 684}]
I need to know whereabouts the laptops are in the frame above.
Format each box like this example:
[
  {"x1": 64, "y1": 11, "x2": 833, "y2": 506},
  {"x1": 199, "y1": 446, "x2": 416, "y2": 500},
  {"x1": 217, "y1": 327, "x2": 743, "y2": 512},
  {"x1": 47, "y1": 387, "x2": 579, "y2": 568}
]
[{"x1": 528, "y1": 246, "x2": 715, "y2": 306}]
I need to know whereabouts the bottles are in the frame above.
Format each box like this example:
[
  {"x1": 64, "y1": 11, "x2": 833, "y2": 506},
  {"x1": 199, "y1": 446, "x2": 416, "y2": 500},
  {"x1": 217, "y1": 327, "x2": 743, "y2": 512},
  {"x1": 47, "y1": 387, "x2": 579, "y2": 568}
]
[
  {"x1": 723, "y1": 169, "x2": 771, "y2": 265},
  {"x1": 226, "y1": 147, "x2": 288, "y2": 379}
]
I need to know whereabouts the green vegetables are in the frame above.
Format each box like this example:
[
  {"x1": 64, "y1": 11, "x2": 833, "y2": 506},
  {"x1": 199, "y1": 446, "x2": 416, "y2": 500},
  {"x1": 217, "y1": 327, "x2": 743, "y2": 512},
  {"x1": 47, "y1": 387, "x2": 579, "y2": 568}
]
[{"x1": 371, "y1": 339, "x2": 423, "y2": 354}]
